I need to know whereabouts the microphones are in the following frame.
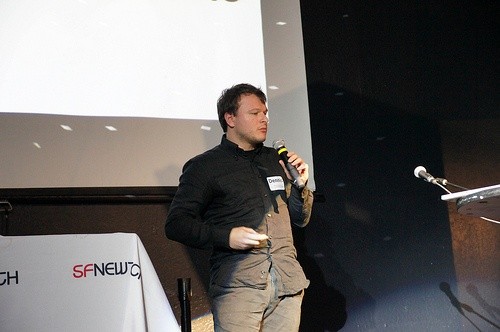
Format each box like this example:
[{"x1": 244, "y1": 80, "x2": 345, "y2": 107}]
[
  {"x1": 273, "y1": 138, "x2": 305, "y2": 190},
  {"x1": 414, "y1": 165, "x2": 437, "y2": 185}
]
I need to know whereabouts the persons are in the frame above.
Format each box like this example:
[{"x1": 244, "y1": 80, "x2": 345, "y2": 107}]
[{"x1": 165, "y1": 84, "x2": 314, "y2": 332}]
